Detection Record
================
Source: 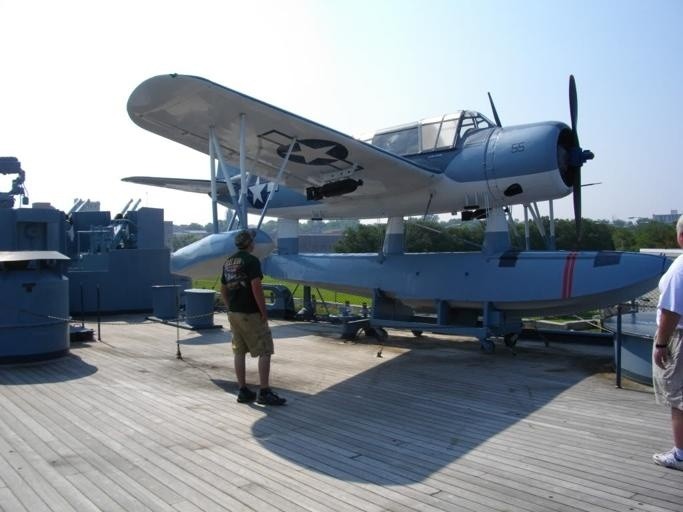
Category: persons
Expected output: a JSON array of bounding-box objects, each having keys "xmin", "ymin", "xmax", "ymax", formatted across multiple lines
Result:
[
  {"xmin": 220, "ymin": 231, "xmax": 287, "ymax": 407},
  {"xmin": 651, "ymin": 214, "xmax": 682, "ymax": 470},
  {"xmin": 344, "ymin": 301, "xmax": 353, "ymax": 315},
  {"xmin": 359, "ymin": 302, "xmax": 371, "ymax": 315}
]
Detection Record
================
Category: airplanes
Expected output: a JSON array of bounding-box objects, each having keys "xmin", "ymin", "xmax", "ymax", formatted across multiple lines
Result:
[{"xmin": 119, "ymin": 70, "xmax": 676, "ymax": 358}]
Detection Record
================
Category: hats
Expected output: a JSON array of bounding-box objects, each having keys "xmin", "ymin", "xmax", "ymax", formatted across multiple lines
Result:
[{"xmin": 234, "ymin": 229, "xmax": 256, "ymax": 249}]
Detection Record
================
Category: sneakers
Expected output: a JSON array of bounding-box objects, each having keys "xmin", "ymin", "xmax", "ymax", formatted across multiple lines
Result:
[
  {"xmin": 652, "ymin": 447, "xmax": 683, "ymax": 471},
  {"xmin": 237, "ymin": 389, "xmax": 257, "ymax": 403},
  {"xmin": 256, "ymin": 391, "xmax": 287, "ymax": 406}
]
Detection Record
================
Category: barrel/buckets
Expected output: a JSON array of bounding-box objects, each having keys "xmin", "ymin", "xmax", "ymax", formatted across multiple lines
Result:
[
  {"xmin": 183, "ymin": 288, "xmax": 216, "ymax": 328},
  {"xmin": 151, "ymin": 285, "xmax": 182, "ymax": 320}
]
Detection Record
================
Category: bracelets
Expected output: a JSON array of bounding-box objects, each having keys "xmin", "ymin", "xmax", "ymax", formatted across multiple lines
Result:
[{"xmin": 655, "ymin": 344, "xmax": 668, "ymax": 348}]
